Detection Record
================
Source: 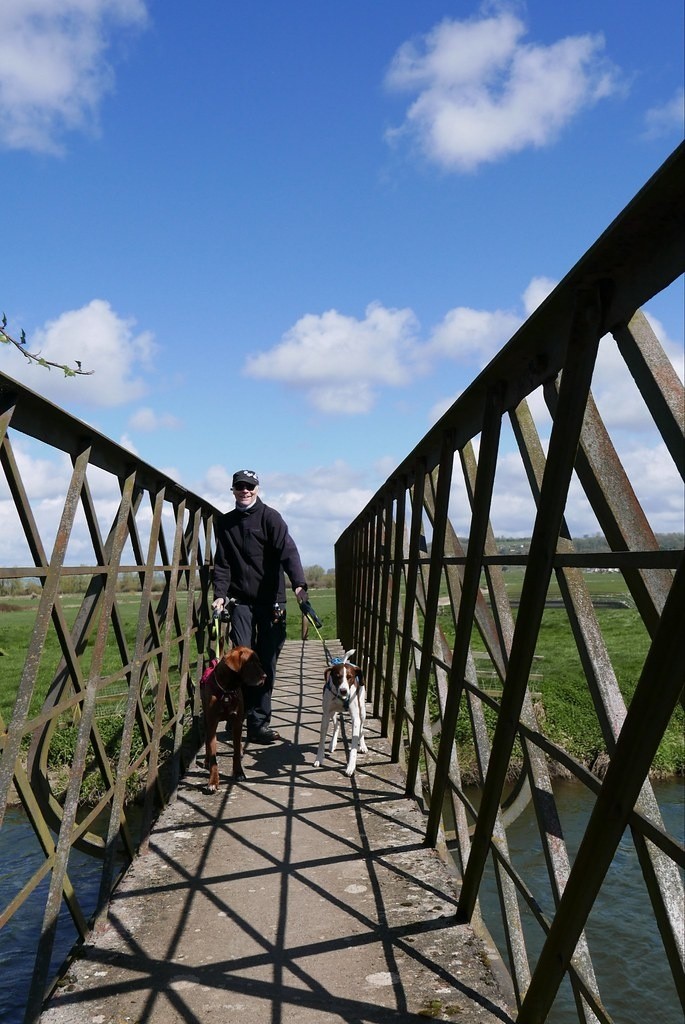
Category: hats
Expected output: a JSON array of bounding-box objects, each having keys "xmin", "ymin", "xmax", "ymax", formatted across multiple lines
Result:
[{"xmin": 232, "ymin": 470, "xmax": 259, "ymax": 485}]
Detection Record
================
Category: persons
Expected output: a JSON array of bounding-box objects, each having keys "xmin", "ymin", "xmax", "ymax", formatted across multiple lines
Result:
[{"xmin": 212, "ymin": 470, "xmax": 309, "ymax": 739}]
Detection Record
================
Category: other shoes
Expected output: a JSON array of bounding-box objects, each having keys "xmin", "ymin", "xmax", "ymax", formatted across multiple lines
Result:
[
  {"xmin": 225, "ymin": 721, "xmax": 235, "ymax": 733},
  {"xmin": 246, "ymin": 723, "xmax": 280, "ymax": 744}
]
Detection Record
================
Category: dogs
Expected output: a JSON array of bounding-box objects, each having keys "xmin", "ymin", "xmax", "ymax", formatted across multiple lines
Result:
[
  {"xmin": 313, "ymin": 649, "xmax": 369, "ymax": 777},
  {"xmin": 200, "ymin": 646, "xmax": 268, "ymax": 793}
]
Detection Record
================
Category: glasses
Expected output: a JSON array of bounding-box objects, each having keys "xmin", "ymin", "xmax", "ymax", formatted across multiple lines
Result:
[{"xmin": 233, "ymin": 483, "xmax": 256, "ymax": 491}]
